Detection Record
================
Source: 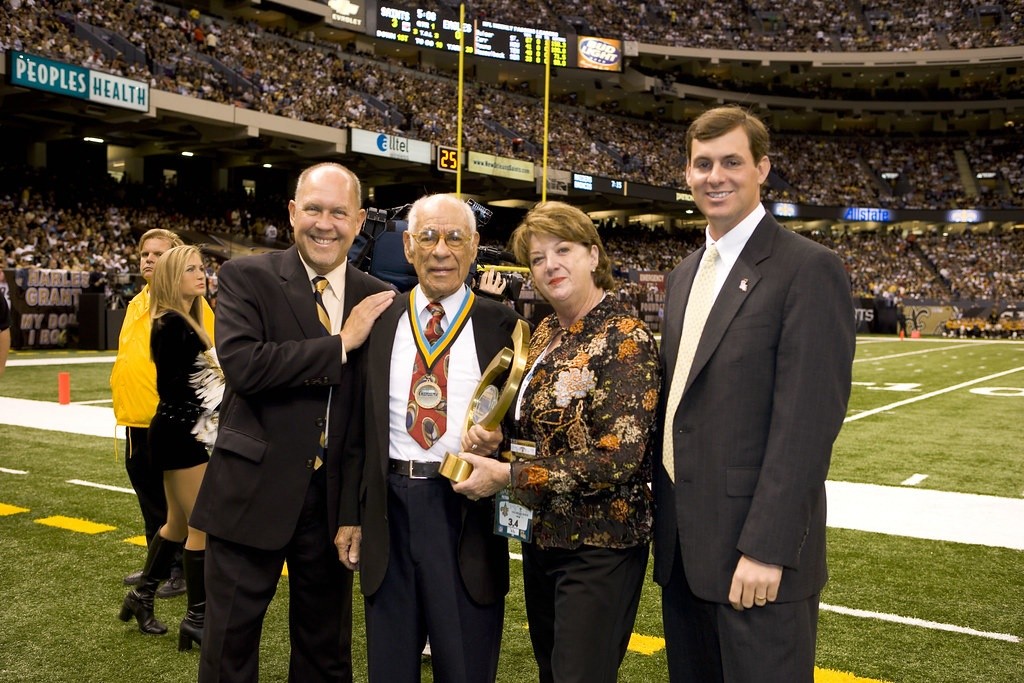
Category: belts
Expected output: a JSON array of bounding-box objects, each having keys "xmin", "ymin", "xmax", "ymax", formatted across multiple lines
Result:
[{"xmin": 388, "ymin": 459, "xmax": 444, "ymax": 480}]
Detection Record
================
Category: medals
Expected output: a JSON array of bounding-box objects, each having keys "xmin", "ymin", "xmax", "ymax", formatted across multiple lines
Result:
[{"xmin": 414, "ymin": 379, "xmax": 442, "ymax": 409}]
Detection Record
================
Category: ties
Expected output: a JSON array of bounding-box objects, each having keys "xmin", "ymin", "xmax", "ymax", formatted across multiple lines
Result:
[
  {"xmin": 662, "ymin": 245, "xmax": 720, "ymax": 483},
  {"xmin": 308, "ymin": 276, "xmax": 331, "ymax": 469},
  {"xmin": 405, "ymin": 301, "xmax": 451, "ymax": 449}
]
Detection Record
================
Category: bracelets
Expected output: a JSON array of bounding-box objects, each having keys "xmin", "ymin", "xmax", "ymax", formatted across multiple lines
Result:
[{"xmin": 508, "ymin": 462, "xmax": 511, "ymax": 488}]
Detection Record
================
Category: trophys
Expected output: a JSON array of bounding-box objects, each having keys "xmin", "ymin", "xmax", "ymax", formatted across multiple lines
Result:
[{"xmin": 437, "ymin": 319, "xmax": 531, "ymax": 482}]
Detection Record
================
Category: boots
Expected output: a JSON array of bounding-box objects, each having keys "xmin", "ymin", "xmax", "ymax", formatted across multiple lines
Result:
[
  {"xmin": 119, "ymin": 524, "xmax": 183, "ymax": 634},
  {"xmin": 178, "ymin": 544, "xmax": 205, "ymax": 651}
]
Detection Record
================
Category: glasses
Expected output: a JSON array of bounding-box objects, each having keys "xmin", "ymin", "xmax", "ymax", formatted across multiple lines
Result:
[{"xmin": 410, "ymin": 229, "xmax": 474, "ymax": 250}]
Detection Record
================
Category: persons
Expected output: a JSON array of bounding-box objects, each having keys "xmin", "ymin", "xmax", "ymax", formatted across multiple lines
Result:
[
  {"xmin": 194, "ymin": 162, "xmax": 400, "ymax": 683},
  {"xmin": 335, "ymin": 189, "xmax": 533, "ymax": 683},
  {"xmin": 110, "ymin": 229, "xmax": 187, "ymax": 599},
  {"xmin": 115, "ymin": 245, "xmax": 226, "ymax": 650},
  {"xmin": 651, "ymin": 108, "xmax": 857, "ymax": 683},
  {"xmin": 448, "ymin": 200, "xmax": 663, "ymax": 683},
  {"xmin": 0, "ymin": 0, "xmax": 1024, "ymax": 377}
]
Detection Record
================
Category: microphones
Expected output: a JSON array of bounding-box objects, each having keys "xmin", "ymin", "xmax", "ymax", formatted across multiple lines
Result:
[{"xmin": 484, "ymin": 247, "xmax": 519, "ymax": 264}]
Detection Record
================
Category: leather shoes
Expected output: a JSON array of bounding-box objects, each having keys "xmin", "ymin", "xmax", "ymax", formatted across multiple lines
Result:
[
  {"xmin": 125, "ymin": 568, "xmax": 145, "ymax": 585},
  {"xmin": 156, "ymin": 577, "xmax": 187, "ymax": 597}
]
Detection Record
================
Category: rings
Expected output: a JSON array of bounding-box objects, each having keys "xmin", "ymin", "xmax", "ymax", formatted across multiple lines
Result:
[
  {"xmin": 754, "ymin": 594, "xmax": 766, "ymax": 601},
  {"xmin": 472, "ymin": 444, "xmax": 477, "ymax": 450}
]
